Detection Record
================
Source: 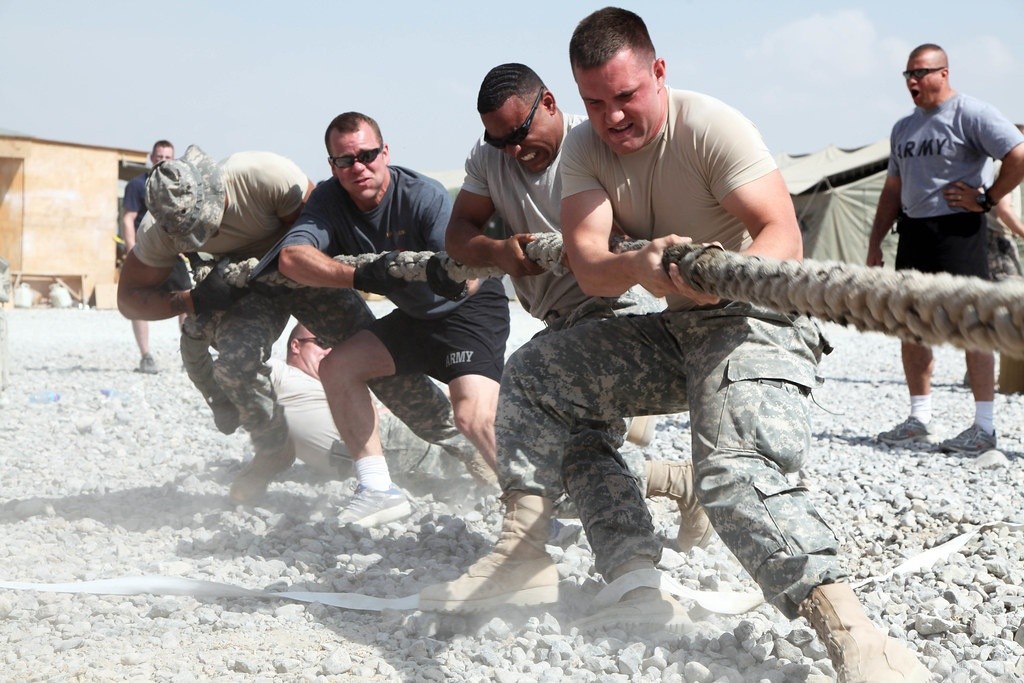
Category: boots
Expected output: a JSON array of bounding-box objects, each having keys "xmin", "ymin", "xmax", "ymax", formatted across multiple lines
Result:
[
  {"xmin": 230, "ymin": 414, "xmax": 298, "ymax": 504},
  {"xmin": 646, "ymin": 461, "xmax": 714, "ymax": 552},
  {"xmin": 188, "ymin": 370, "xmax": 238, "ymax": 436},
  {"xmin": 453, "ymin": 449, "xmax": 502, "ymax": 503},
  {"xmin": 797, "ymin": 583, "xmax": 932, "ymax": 683},
  {"xmin": 419, "ymin": 491, "xmax": 561, "ymax": 616},
  {"xmin": 625, "ymin": 415, "xmax": 659, "ymax": 446},
  {"xmin": 564, "ymin": 555, "xmax": 693, "ymax": 639}
]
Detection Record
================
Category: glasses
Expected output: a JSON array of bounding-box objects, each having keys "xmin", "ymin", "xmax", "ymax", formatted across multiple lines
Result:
[
  {"xmin": 329, "ymin": 145, "xmax": 384, "ymax": 167},
  {"xmin": 902, "ymin": 67, "xmax": 945, "ymax": 80},
  {"xmin": 484, "ymin": 87, "xmax": 544, "ymax": 149},
  {"xmin": 289, "ymin": 336, "xmax": 330, "ymax": 350}
]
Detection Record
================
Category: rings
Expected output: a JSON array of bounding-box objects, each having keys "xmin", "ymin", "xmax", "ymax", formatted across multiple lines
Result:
[{"xmin": 957, "ymin": 194, "xmax": 963, "ymax": 201}]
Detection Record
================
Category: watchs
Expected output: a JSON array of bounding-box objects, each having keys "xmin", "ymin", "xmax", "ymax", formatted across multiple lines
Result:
[{"xmin": 976, "ymin": 185, "xmax": 991, "ymax": 213}]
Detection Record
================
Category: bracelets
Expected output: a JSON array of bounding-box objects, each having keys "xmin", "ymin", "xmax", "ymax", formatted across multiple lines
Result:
[{"xmin": 985, "ymin": 188, "xmax": 1000, "ymax": 207}]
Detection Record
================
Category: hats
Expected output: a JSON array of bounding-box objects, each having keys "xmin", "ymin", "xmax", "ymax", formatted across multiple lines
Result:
[{"xmin": 145, "ymin": 143, "xmax": 225, "ymax": 253}]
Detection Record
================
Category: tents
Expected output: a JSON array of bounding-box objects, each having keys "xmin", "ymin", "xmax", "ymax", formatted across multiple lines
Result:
[{"xmin": 741, "ymin": 140, "xmax": 900, "ymax": 271}]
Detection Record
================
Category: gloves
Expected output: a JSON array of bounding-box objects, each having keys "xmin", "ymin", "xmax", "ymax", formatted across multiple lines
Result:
[
  {"xmin": 354, "ymin": 250, "xmax": 408, "ymax": 296},
  {"xmin": 189, "ymin": 257, "xmax": 251, "ymax": 315},
  {"xmin": 426, "ymin": 251, "xmax": 466, "ymax": 299}
]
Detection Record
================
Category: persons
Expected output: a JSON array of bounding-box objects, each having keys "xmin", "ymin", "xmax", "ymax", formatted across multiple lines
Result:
[
  {"xmin": 866, "ymin": 43, "xmax": 1024, "ymax": 457},
  {"xmin": 419, "ymin": 6, "xmax": 937, "ymax": 682},
  {"xmin": 245, "ymin": 112, "xmax": 510, "ymax": 531},
  {"xmin": 261, "ymin": 319, "xmax": 718, "ymax": 553},
  {"xmin": 444, "ymin": 63, "xmax": 703, "ymax": 638},
  {"xmin": 118, "ymin": 140, "xmax": 316, "ymax": 499}
]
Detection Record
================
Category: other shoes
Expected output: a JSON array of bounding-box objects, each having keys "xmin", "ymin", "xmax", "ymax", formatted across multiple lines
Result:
[{"xmin": 139, "ymin": 352, "xmax": 159, "ymax": 374}]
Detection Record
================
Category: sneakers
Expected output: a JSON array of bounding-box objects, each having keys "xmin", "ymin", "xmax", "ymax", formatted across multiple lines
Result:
[
  {"xmin": 544, "ymin": 517, "xmax": 582, "ymax": 551},
  {"xmin": 337, "ymin": 480, "xmax": 410, "ymax": 528},
  {"xmin": 878, "ymin": 416, "xmax": 938, "ymax": 445},
  {"xmin": 939, "ymin": 425, "xmax": 997, "ymax": 454}
]
